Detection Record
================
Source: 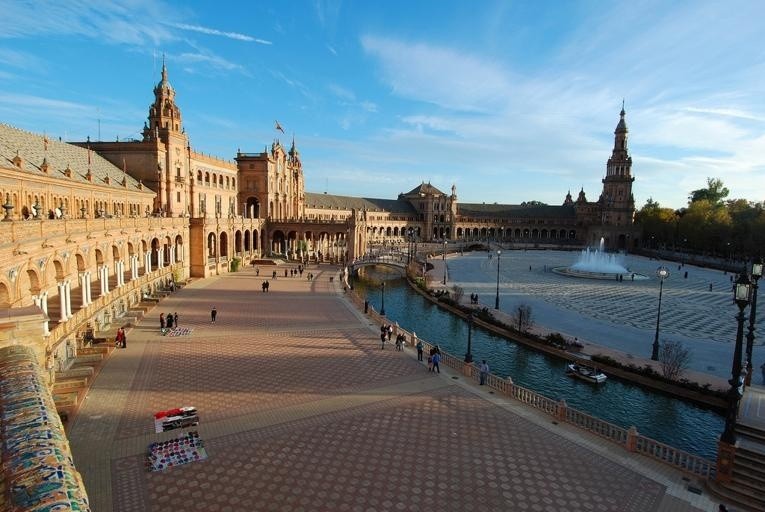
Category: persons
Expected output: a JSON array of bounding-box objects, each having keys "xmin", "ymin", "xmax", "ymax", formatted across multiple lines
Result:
[
  {"xmin": 473, "ymin": 295, "xmax": 479, "ymax": 304},
  {"xmin": 169, "ymin": 281, "xmax": 174, "ymax": 291},
  {"xmin": 574, "ymin": 337, "xmax": 580, "ymax": 346},
  {"xmin": 211, "ymin": 307, "xmax": 217, "ymax": 324},
  {"xmin": 160, "ymin": 312, "xmax": 179, "ymax": 328},
  {"xmin": 256, "ymin": 260, "xmax": 313, "ymax": 293},
  {"xmin": 115, "ymin": 328, "xmax": 122, "ymax": 346},
  {"xmin": 479, "ymin": 360, "xmax": 489, "ymax": 385},
  {"xmin": 529, "ymin": 266, "xmax": 531, "ymax": 272},
  {"xmin": 615, "ymin": 257, "xmax": 739, "ymax": 292},
  {"xmin": 471, "ymin": 293, "xmax": 474, "ymax": 304},
  {"xmin": 573, "ymin": 359, "xmax": 601, "ymax": 376},
  {"xmin": 381, "ymin": 323, "xmax": 441, "ymax": 373},
  {"xmin": 121, "ymin": 327, "xmax": 126, "ymax": 348}
]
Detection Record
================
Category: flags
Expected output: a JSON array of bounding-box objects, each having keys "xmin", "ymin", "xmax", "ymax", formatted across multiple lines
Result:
[{"xmin": 275, "ymin": 121, "xmax": 285, "ymax": 134}]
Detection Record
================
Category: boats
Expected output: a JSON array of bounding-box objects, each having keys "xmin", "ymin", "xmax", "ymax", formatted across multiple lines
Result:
[{"xmin": 566, "ymin": 360, "xmax": 609, "ymax": 384}]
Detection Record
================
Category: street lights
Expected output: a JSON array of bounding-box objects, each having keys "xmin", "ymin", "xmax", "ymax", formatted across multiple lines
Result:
[
  {"xmin": 444, "ymin": 241, "xmax": 447, "ymax": 285},
  {"xmin": 501, "ymin": 226, "xmax": 504, "ymax": 247},
  {"xmin": 570, "ymin": 231, "xmax": 574, "ymax": 251},
  {"xmin": 494, "ymin": 249, "xmax": 504, "ymax": 309},
  {"xmin": 465, "ymin": 304, "xmax": 475, "ymax": 365},
  {"xmin": 680, "ymin": 233, "xmax": 688, "ymax": 266},
  {"xmin": 460, "ymin": 231, "xmax": 464, "ymax": 257},
  {"xmin": 651, "ymin": 266, "xmax": 669, "ymax": 362},
  {"xmin": 747, "ymin": 248, "xmax": 763, "ymax": 372},
  {"xmin": 624, "ymin": 234, "xmax": 630, "ymax": 256},
  {"xmin": 406, "ymin": 227, "xmax": 419, "ymax": 262},
  {"xmin": 649, "ymin": 234, "xmax": 656, "ymax": 260},
  {"xmin": 525, "ymin": 231, "xmax": 528, "ymax": 251},
  {"xmin": 442, "ymin": 232, "xmax": 446, "ymax": 260},
  {"xmin": 725, "ymin": 237, "xmax": 732, "ymax": 274},
  {"xmin": 379, "ymin": 282, "xmax": 388, "ymax": 315},
  {"xmin": 720, "ymin": 261, "xmax": 755, "ymax": 444},
  {"xmin": 486, "ymin": 227, "xmax": 490, "ymax": 252}
]
